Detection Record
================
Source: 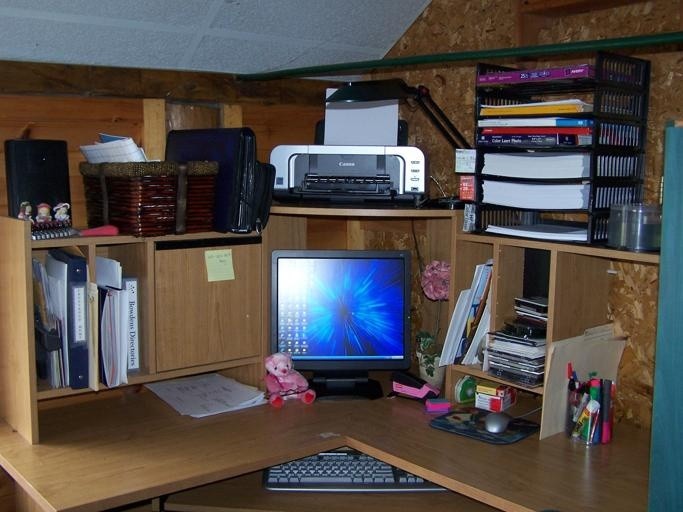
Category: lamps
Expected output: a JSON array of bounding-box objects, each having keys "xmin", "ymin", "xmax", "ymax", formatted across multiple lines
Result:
[{"xmin": 325, "ymin": 77, "xmax": 472, "ymax": 149}]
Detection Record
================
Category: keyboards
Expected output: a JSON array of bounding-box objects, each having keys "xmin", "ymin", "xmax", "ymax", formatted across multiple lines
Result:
[{"xmin": 261, "ymin": 452, "xmax": 447, "ymax": 491}]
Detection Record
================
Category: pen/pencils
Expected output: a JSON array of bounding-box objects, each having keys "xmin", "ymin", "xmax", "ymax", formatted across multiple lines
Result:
[{"xmin": 567, "ymin": 363, "xmax": 615, "ymax": 444}]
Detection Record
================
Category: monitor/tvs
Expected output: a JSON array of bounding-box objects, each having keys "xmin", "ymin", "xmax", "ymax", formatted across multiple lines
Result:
[{"xmin": 272, "ymin": 249, "xmax": 411, "ymax": 399}]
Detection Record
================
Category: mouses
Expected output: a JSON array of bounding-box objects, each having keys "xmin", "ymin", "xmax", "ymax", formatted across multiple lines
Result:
[{"xmin": 485, "ymin": 412, "xmax": 512, "ymax": 434}]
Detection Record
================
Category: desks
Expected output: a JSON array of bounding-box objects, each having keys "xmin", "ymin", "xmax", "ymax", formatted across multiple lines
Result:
[{"xmin": 0, "ymin": 373, "xmax": 651, "ymax": 512}]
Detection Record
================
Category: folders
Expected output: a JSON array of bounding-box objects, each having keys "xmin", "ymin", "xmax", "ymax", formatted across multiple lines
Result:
[{"xmin": 49, "ymin": 248, "xmax": 86, "ymax": 388}]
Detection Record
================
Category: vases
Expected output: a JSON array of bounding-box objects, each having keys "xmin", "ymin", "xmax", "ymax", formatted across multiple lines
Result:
[{"xmin": 416, "ymin": 351, "xmax": 443, "ymax": 390}]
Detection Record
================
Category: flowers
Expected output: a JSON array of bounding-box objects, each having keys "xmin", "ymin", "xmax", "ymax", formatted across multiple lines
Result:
[{"xmin": 415, "ymin": 258, "xmax": 451, "ymax": 378}]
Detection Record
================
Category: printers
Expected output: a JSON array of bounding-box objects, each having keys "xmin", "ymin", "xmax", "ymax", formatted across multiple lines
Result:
[{"xmin": 269, "ymin": 118, "xmax": 430, "ymax": 209}]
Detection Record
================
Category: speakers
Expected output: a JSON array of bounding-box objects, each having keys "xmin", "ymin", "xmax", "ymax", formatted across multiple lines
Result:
[{"xmin": 3, "ymin": 138, "xmax": 73, "ymax": 228}]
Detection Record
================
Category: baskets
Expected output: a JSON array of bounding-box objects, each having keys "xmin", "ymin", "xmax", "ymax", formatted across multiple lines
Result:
[{"xmin": 80, "ymin": 160, "xmax": 218, "ymax": 238}]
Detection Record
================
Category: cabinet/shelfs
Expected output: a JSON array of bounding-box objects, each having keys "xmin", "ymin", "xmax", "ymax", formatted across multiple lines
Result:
[
  {"xmin": 444, "ymin": 231, "xmax": 610, "ymax": 430},
  {"xmin": 2, "ymin": 215, "xmax": 152, "ymax": 400},
  {"xmin": 152, "ymin": 241, "xmax": 262, "ymax": 383}
]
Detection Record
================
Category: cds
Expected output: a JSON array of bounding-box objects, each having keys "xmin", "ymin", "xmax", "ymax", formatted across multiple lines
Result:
[{"xmin": 607, "ymin": 219, "xmax": 661, "ymax": 250}]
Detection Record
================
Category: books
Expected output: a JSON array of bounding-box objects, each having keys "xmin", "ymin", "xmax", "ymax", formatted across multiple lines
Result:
[
  {"xmin": 30, "ymin": 227, "xmax": 73, "ymax": 240},
  {"xmin": 438, "ymin": 258, "xmax": 547, "ymax": 389},
  {"xmin": 144, "ymin": 371, "xmax": 268, "ymax": 419},
  {"xmin": 78, "ymin": 131, "xmax": 161, "ymax": 163},
  {"xmin": 32, "ymin": 245, "xmax": 141, "ymax": 392},
  {"xmin": 477, "ymin": 97, "xmax": 639, "ymax": 242}
]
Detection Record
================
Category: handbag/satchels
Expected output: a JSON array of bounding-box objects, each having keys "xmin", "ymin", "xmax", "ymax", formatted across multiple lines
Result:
[
  {"xmin": 254, "ymin": 159, "xmax": 276, "ymax": 230},
  {"xmin": 164, "ymin": 127, "xmax": 255, "ymax": 234}
]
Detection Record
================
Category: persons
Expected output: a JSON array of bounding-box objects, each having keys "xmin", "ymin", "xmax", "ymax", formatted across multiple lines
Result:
[
  {"xmin": 17, "ymin": 198, "xmax": 35, "ymax": 224},
  {"xmin": 35, "ymin": 201, "xmax": 52, "ymax": 228},
  {"xmin": 53, "ymin": 201, "xmax": 72, "ymax": 227}
]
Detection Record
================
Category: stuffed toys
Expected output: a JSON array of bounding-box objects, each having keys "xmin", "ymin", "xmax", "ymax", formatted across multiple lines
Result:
[{"xmin": 263, "ymin": 351, "xmax": 316, "ymax": 407}]
